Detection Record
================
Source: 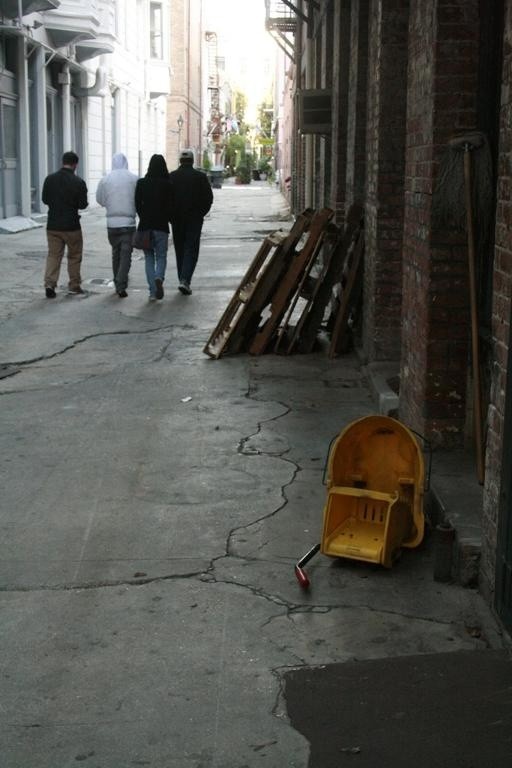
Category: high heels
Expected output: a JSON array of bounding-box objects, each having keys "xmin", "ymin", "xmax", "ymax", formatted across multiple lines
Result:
[{"xmin": 116, "ymin": 289, "xmax": 127, "ymax": 297}]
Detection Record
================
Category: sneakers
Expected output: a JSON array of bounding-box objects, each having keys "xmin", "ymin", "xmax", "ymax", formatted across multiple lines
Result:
[
  {"xmin": 68, "ymin": 286, "xmax": 83, "ymax": 294},
  {"xmin": 177, "ymin": 285, "xmax": 192, "ymax": 295},
  {"xmin": 147, "ymin": 277, "xmax": 164, "ymax": 302},
  {"xmin": 45, "ymin": 288, "xmax": 56, "ymax": 298}
]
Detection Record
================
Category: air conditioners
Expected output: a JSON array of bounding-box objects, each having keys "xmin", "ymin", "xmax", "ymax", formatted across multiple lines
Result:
[{"xmin": 295, "ymin": 88, "xmax": 331, "ymax": 135}]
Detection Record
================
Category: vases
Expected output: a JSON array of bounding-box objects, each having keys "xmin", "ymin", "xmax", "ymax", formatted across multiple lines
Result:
[{"xmin": 253, "ymin": 170, "xmax": 261, "ymax": 180}]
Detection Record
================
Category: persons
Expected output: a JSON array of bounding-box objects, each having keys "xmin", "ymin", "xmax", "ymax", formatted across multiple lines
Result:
[
  {"xmin": 40, "ymin": 150, "xmax": 88, "ymax": 298},
  {"xmin": 94, "ymin": 153, "xmax": 139, "ymax": 298},
  {"xmin": 134, "ymin": 153, "xmax": 171, "ymax": 301},
  {"xmin": 162, "ymin": 149, "xmax": 215, "ymax": 294}
]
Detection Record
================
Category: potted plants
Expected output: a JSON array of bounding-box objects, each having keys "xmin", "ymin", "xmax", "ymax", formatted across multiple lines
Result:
[
  {"xmin": 235, "ymin": 155, "xmax": 251, "ymax": 184},
  {"xmin": 259, "ymin": 154, "xmax": 271, "ymax": 181}
]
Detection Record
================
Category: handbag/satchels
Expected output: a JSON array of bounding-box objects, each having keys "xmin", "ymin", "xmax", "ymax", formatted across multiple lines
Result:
[{"xmin": 131, "ymin": 231, "xmax": 150, "ymax": 249}]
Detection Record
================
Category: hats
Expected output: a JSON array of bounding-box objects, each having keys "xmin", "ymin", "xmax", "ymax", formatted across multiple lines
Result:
[{"xmin": 179, "ymin": 150, "xmax": 193, "ymax": 160}]
